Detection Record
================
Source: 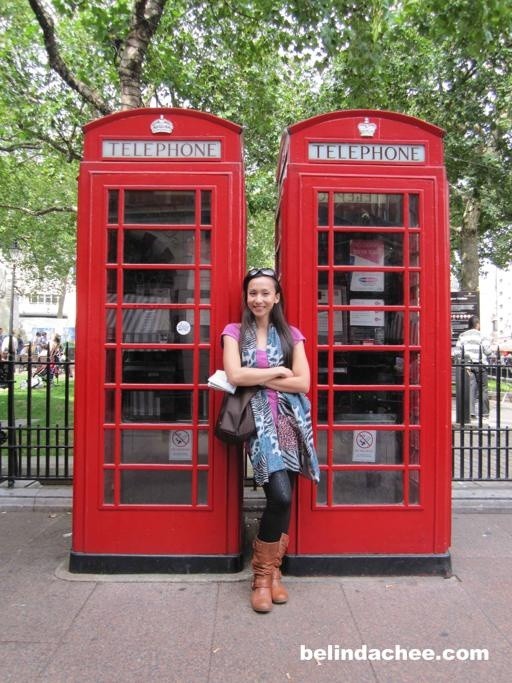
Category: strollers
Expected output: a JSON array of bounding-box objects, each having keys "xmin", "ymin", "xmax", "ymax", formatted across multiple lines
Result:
[{"xmin": 31, "ymin": 351, "xmax": 60, "ymax": 386}]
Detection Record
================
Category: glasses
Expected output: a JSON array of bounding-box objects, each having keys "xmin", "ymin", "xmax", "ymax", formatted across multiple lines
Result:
[{"xmin": 248, "ymin": 268, "xmax": 275, "ymax": 278}]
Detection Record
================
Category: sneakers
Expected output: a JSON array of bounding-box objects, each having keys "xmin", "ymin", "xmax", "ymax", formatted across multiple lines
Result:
[{"xmin": 470, "ymin": 415, "xmax": 490, "ymax": 429}]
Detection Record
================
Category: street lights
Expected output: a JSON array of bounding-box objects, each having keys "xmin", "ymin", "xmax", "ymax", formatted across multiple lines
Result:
[{"xmin": 9, "ymin": 237, "xmax": 26, "ymax": 357}]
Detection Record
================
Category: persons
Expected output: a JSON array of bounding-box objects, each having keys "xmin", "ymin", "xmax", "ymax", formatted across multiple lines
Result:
[
  {"xmin": 0, "ymin": 327, "xmax": 71, "ymax": 387},
  {"xmin": 456, "ymin": 316, "xmax": 492, "ymax": 420},
  {"xmin": 220, "ymin": 268, "xmax": 312, "ymax": 613}
]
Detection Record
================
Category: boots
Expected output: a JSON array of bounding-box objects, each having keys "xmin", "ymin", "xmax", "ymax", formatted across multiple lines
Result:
[
  {"xmin": 250, "ymin": 535, "xmax": 280, "ymax": 612},
  {"xmin": 270, "ymin": 533, "xmax": 290, "ymax": 604}
]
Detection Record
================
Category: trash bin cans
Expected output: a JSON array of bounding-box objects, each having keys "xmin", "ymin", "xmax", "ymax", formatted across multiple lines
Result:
[
  {"xmin": 167, "ymin": 420, "xmax": 208, "ymax": 504},
  {"xmin": 336, "ymin": 413, "xmax": 397, "ymax": 505}
]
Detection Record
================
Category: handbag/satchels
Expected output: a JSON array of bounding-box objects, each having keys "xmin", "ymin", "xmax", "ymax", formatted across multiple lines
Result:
[{"xmin": 213, "ymin": 385, "xmax": 261, "ymax": 444}]
